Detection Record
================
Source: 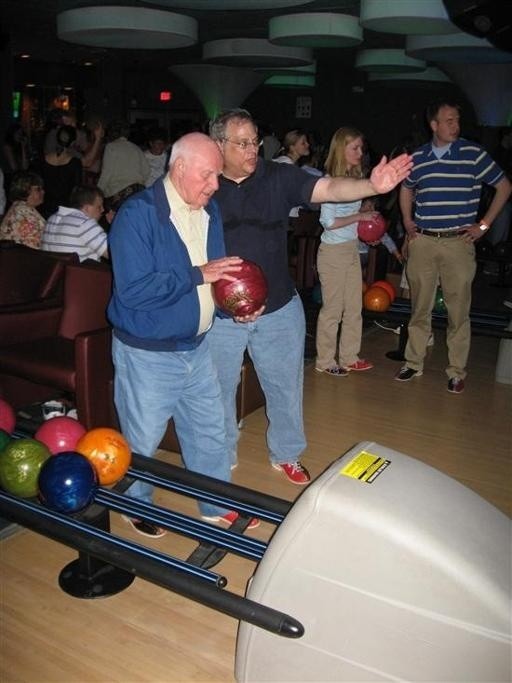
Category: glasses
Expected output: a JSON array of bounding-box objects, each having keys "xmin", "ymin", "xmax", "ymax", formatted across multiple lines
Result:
[{"xmin": 221, "ymin": 135, "xmax": 261, "ymax": 148}]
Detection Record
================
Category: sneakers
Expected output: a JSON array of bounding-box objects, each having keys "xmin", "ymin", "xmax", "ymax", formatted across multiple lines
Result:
[
  {"xmin": 120, "ymin": 512, "xmax": 169, "ymax": 539},
  {"xmin": 314, "ymin": 362, "xmax": 350, "ymax": 377},
  {"xmin": 372, "ymin": 317, "xmax": 404, "ymax": 335},
  {"xmin": 340, "ymin": 357, "xmax": 376, "ymax": 371},
  {"xmin": 425, "ymin": 331, "xmax": 435, "ymax": 347},
  {"xmin": 201, "ymin": 507, "xmax": 262, "ymax": 530},
  {"xmin": 392, "ymin": 365, "xmax": 424, "ymax": 382},
  {"xmin": 446, "ymin": 374, "xmax": 465, "ymax": 394},
  {"xmin": 270, "ymin": 458, "xmax": 312, "ymax": 484}
]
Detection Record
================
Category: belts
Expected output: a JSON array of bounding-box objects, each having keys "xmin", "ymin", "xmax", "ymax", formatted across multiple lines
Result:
[{"xmin": 413, "ymin": 224, "xmax": 469, "ymax": 239}]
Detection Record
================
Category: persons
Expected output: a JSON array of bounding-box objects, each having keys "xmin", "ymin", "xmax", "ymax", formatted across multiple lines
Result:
[{"xmin": 1, "ymin": 99, "xmax": 511, "ymax": 540}]
